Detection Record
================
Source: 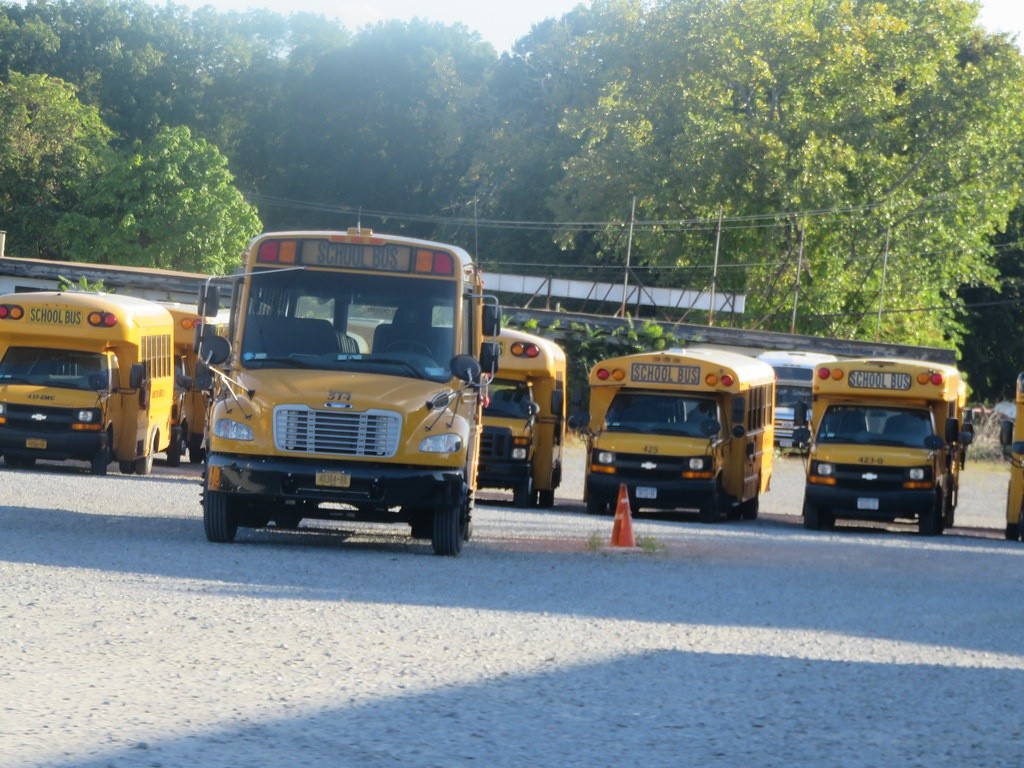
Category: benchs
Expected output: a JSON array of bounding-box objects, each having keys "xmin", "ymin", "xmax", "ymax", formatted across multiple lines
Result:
[
  {"xmin": 17, "ymin": 355, "xmax": 77, "ymax": 376},
  {"xmin": 243, "ymin": 315, "xmax": 360, "ymax": 355}
]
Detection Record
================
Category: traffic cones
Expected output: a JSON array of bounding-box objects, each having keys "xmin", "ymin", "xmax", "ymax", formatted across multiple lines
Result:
[{"xmin": 611, "ymin": 482, "xmax": 635, "ymax": 548}]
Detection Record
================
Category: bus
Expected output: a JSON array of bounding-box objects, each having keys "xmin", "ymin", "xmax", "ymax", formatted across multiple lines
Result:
[
  {"xmin": 755, "ymin": 350, "xmax": 838, "ymax": 450},
  {"xmin": 473, "ymin": 326, "xmax": 566, "ymax": 508},
  {"xmin": 582, "ymin": 347, "xmax": 778, "ymax": 523},
  {"xmin": 193, "ymin": 228, "xmax": 502, "ymax": 557},
  {"xmin": 998, "ymin": 374, "xmax": 1024, "ymax": 542},
  {"xmin": 0, "ymin": 291, "xmax": 174, "ymax": 476},
  {"xmin": 801, "ymin": 359, "xmax": 967, "ymax": 536},
  {"xmin": 150, "ymin": 302, "xmax": 228, "ymax": 463}
]
